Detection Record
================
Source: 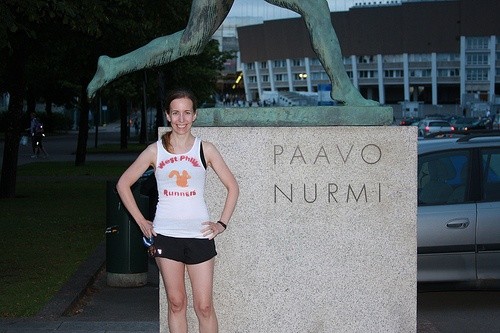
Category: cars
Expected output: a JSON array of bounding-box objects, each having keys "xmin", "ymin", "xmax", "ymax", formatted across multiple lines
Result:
[
  {"xmin": 417, "ymin": 130, "xmax": 500, "ymax": 293},
  {"xmin": 410, "ymin": 118, "xmax": 454, "ymax": 139}
]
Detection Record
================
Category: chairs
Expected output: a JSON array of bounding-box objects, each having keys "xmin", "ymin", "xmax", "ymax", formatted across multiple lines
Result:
[{"xmin": 418, "ymin": 156, "xmax": 470, "ymax": 205}]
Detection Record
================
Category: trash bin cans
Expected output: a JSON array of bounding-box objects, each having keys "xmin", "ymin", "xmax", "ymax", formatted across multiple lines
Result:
[{"xmin": 103, "ymin": 166, "xmax": 158, "ymax": 288}]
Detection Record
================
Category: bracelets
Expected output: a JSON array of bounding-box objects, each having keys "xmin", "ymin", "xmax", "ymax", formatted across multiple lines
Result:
[{"xmin": 217, "ymin": 221, "xmax": 227, "ymax": 229}]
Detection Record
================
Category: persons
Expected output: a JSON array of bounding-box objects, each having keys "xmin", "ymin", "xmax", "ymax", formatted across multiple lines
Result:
[
  {"xmin": 213, "ymin": 91, "xmax": 278, "ymax": 109},
  {"xmin": 26, "ymin": 117, "xmax": 50, "ymax": 159},
  {"xmin": 115, "ymin": 90, "xmax": 239, "ymax": 333},
  {"xmin": 133, "ymin": 117, "xmax": 141, "ymax": 136},
  {"xmin": 88, "ymin": 1, "xmax": 379, "ymax": 106}
]
